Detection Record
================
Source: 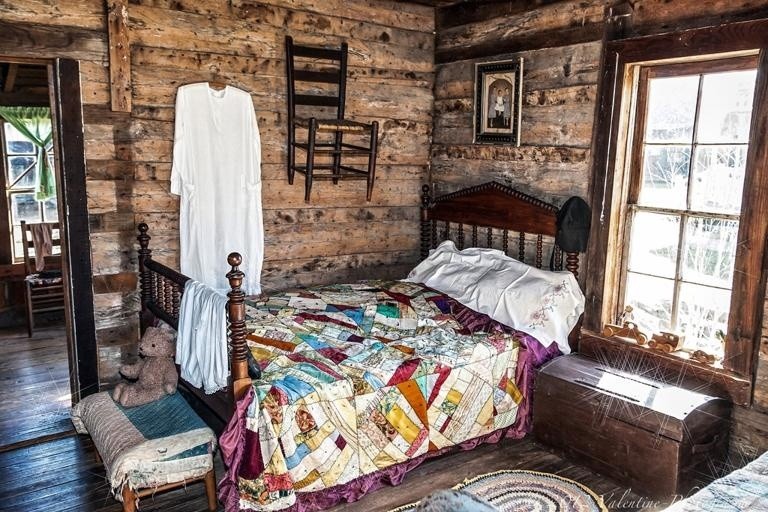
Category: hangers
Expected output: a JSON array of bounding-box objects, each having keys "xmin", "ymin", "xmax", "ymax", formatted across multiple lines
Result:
[{"xmin": 173, "ymin": 63, "xmax": 251, "ymax": 100}]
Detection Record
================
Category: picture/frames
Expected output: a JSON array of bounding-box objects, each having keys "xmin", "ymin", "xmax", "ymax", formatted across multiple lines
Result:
[{"xmin": 472, "ymin": 58, "xmax": 525, "ymax": 146}]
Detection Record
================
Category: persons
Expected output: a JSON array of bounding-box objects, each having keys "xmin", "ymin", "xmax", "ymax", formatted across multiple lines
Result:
[{"xmin": 494, "ymin": 88, "xmax": 511, "ymax": 129}]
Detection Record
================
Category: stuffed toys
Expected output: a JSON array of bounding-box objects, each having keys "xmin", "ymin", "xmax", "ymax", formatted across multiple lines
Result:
[{"xmin": 113, "ymin": 325, "xmax": 178, "ymax": 408}]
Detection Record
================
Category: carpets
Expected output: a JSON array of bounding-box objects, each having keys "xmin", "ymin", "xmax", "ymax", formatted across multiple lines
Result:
[{"xmin": 392, "ymin": 470, "xmax": 609, "ymax": 512}]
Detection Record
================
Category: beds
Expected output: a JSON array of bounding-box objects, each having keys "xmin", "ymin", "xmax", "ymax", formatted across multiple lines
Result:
[{"xmin": 119, "ymin": 181, "xmax": 593, "ymax": 512}]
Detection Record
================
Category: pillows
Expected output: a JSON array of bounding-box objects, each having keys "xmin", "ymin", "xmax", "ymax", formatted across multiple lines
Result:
[{"xmin": 398, "ymin": 239, "xmax": 586, "ymax": 354}]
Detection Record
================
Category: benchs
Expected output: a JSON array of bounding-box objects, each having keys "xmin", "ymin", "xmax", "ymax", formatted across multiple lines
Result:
[{"xmin": 73, "ymin": 383, "xmax": 217, "ymax": 511}]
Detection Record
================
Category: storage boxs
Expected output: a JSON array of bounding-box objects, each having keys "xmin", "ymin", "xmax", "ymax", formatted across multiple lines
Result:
[{"xmin": 533, "ymin": 353, "xmax": 733, "ymax": 502}]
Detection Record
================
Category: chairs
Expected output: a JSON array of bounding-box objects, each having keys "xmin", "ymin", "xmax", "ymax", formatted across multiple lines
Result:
[
  {"xmin": 20, "ymin": 220, "xmax": 66, "ymax": 337},
  {"xmin": 284, "ymin": 36, "xmax": 380, "ymax": 202}
]
